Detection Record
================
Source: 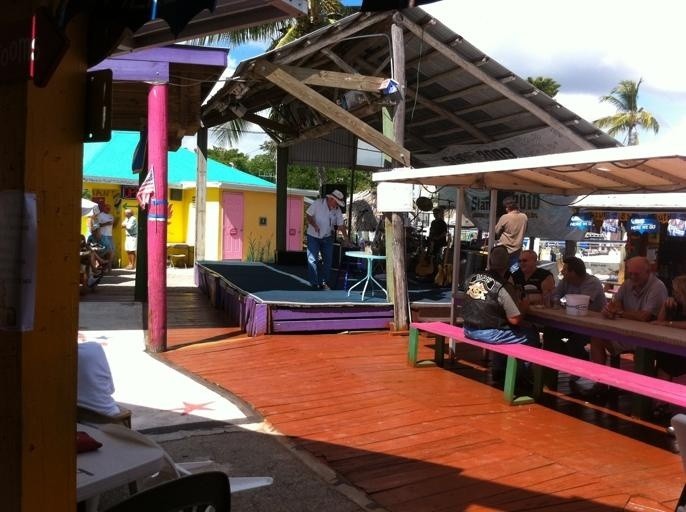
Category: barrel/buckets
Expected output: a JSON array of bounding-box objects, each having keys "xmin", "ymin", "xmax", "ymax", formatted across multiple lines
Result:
[{"xmin": 562, "ymin": 294, "xmax": 591, "ymax": 316}]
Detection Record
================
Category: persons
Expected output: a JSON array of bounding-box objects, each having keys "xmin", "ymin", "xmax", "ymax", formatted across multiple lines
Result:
[
  {"xmin": 427, "ymin": 208, "xmax": 448, "ymax": 265},
  {"xmin": 121, "ymin": 208, "xmax": 138, "ymax": 270},
  {"xmin": 80, "ymin": 205, "xmax": 115, "ymax": 296},
  {"xmin": 305, "ymin": 189, "xmax": 353, "ymax": 291},
  {"xmin": 494, "ymin": 195, "xmax": 529, "ymax": 268}
]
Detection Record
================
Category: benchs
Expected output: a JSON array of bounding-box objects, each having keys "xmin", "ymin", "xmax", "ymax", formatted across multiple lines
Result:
[{"xmin": 405, "ymin": 319, "xmax": 686, "ymax": 437}]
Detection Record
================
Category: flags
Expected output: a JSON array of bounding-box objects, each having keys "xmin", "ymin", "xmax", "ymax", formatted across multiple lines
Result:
[{"xmin": 136, "ymin": 165, "xmax": 156, "ymax": 210}]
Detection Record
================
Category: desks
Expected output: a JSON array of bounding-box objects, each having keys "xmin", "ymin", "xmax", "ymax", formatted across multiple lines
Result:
[
  {"xmin": 342, "ymin": 249, "xmax": 390, "ymax": 302},
  {"xmin": 451, "ymin": 289, "xmax": 686, "ymax": 410},
  {"xmin": 72, "ymin": 419, "xmax": 166, "ymax": 511}
]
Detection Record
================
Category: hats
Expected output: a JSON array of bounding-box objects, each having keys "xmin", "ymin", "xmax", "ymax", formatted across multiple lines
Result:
[{"xmin": 326, "ymin": 190, "xmax": 346, "ymax": 208}]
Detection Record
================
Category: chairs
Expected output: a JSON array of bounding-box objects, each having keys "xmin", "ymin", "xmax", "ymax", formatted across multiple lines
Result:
[
  {"xmin": 101, "ymin": 466, "xmax": 232, "ymax": 512},
  {"xmin": 166, "ymin": 243, "xmax": 190, "ymax": 272},
  {"xmin": 75, "ymin": 339, "xmax": 141, "ymax": 497}
]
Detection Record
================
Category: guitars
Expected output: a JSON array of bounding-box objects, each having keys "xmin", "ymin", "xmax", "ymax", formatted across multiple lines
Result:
[
  {"xmin": 434, "ymin": 233, "xmax": 453, "ymax": 286},
  {"xmin": 415, "ymin": 237, "xmax": 435, "ymax": 276}
]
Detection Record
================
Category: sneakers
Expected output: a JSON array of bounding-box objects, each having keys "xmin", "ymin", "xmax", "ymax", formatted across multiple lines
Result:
[{"xmin": 320, "ymin": 284, "xmax": 333, "ymax": 290}]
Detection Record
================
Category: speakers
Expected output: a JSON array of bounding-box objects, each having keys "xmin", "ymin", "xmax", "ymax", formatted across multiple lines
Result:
[
  {"xmin": 82, "ymin": 69, "xmax": 113, "ymax": 142},
  {"xmin": 319, "ymin": 184, "xmax": 347, "ymax": 214}
]
